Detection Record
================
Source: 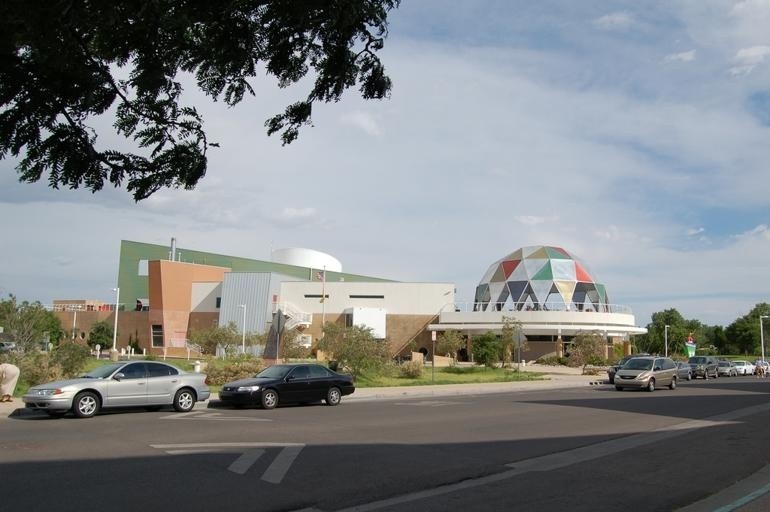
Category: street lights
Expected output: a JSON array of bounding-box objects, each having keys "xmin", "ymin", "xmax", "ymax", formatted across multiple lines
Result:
[
  {"xmin": 759, "ymin": 314, "xmax": 769, "ymax": 361},
  {"xmin": 112, "ymin": 288, "xmax": 120, "ymax": 350},
  {"xmin": 70, "ymin": 305, "xmax": 82, "ymax": 342},
  {"xmin": 237, "ymin": 304, "xmax": 247, "ymax": 354},
  {"xmin": 664, "ymin": 324, "xmax": 671, "ymax": 357}
]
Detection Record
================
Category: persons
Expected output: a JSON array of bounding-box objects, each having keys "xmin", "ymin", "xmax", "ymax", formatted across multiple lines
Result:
[
  {"xmin": 1, "ymin": 362, "xmax": 22, "ymax": 402},
  {"xmin": 754, "ymin": 356, "xmax": 766, "ymax": 379}
]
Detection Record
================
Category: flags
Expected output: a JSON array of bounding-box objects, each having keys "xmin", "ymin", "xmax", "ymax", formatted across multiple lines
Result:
[{"xmin": 314, "ymin": 270, "xmax": 325, "ymax": 282}]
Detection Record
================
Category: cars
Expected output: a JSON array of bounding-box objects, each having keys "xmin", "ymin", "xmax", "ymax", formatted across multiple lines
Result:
[
  {"xmin": 606, "ymin": 352, "xmax": 770, "ymax": 391},
  {"xmin": 0, "ymin": 341, "xmax": 24, "ymax": 353},
  {"xmin": 22, "ymin": 359, "xmax": 212, "ymax": 420},
  {"xmin": 219, "ymin": 361, "xmax": 355, "ymax": 409}
]
